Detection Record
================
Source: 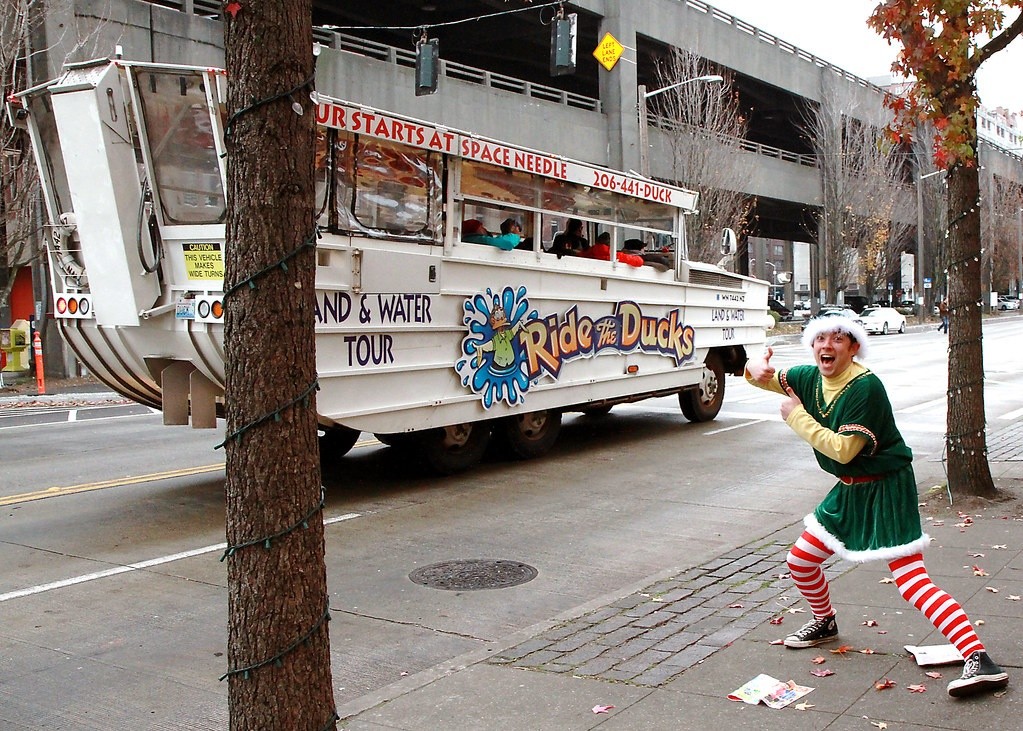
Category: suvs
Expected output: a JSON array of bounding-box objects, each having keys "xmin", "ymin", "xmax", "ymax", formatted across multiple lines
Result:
[{"xmin": 998, "ymin": 295, "xmax": 1020, "ymax": 307}]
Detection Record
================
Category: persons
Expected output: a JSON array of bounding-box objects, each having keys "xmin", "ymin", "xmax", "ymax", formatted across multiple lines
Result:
[
  {"xmin": 936, "ymin": 297, "xmax": 950, "ymax": 335},
  {"xmin": 499, "ymin": 218, "xmax": 534, "ymax": 252},
  {"xmin": 742, "ymin": 315, "xmax": 1009, "ymax": 699},
  {"xmin": 623, "ymin": 239, "xmax": 647, "ymax": 253},
  {"xmin": 580, "ymin": 231, "xmax": 643, "ymax": 269},
  {"xmin": 552, "ymin": 218, "xmax": 591, "ymax": 258},
  {"xmin": 462, "ymin": 218, "xmax": 524, "ymax": 251}
]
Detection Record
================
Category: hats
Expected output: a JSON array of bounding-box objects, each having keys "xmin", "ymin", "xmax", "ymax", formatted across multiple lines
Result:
[
  {"xmin": 501, "ymin": 218, "xmax": 515, "ymax": 231},
  {"xmin": 803, "ymin": 310, "xmax": 870, "ymax": 360},
  {"xmin": 624, "ymin": 239, "xmax": 647, "ymax": 250},
  {"xmin": 462, "ymin": 219, "xmax": 483, "ymax": 234}
]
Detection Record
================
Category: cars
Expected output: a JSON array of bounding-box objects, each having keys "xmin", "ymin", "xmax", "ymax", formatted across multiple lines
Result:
[
  {"xmin": 801, "ymin": 304, "xmax": 864, "ymax": 331},
  {"xmin": 997, "ymin": 299, "xmax": 1018, "ymax": 311},
  {"xmin": 770, "ymin": 298, "xmax": 941, "ymax": 319},
  {"xmin": 859, "ymin": 307, "xmax": 906, "ymax": 335}
]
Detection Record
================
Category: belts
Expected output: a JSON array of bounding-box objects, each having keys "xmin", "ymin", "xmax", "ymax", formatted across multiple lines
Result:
[{"xmin": 837, "ymin": 474, "xmax": 886, "ymax": 485}]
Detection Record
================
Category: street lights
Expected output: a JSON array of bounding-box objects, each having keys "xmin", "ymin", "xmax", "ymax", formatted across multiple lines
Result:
[{"xmin": 637, "ymin": 75, "xmax": 723, "ymax": 178}]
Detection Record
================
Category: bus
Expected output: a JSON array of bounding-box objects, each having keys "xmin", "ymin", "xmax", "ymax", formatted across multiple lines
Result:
[{"xmin": 5, "ymin": 56, "xmax": 794, "ymax": 473}]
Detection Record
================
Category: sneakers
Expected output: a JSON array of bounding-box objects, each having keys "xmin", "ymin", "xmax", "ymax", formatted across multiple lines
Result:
[
  {"xmin": 783, "ymin": 608, "xmax": 838, "ymax": 647},
  {"xmin": 947, "ymin": 652, "xmax": 1010, "ymax": 695}
]
